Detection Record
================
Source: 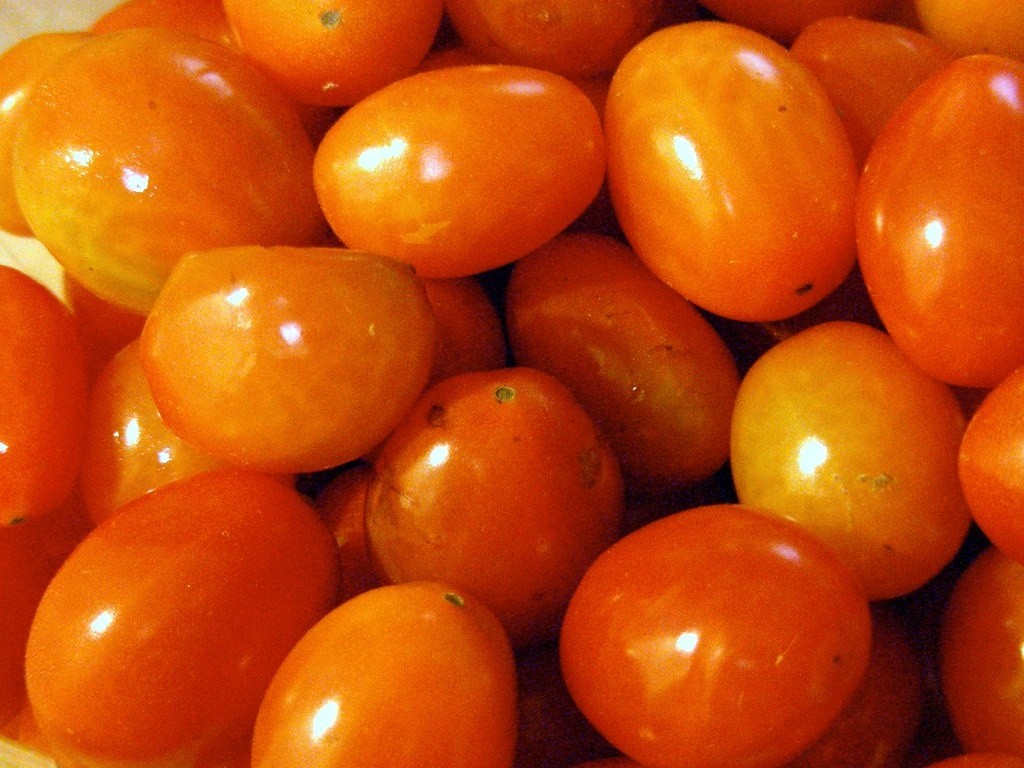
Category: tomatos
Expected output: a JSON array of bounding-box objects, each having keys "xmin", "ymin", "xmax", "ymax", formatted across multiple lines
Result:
[{"xmin": 0, "ymin": 0, "xmax": 1024, "ymax": 768}]
